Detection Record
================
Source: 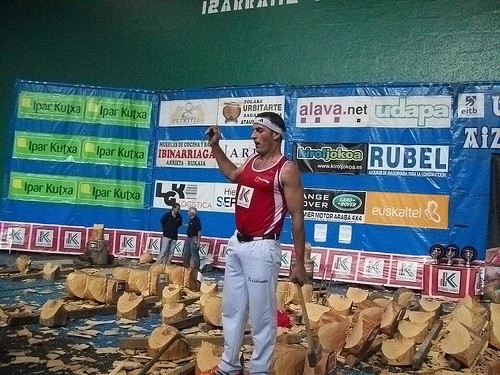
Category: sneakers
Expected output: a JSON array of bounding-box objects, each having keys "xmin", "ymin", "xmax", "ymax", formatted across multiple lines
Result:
[{"xmin": 197, "ymin": 365, "xmax": 240, "ymax": 375}]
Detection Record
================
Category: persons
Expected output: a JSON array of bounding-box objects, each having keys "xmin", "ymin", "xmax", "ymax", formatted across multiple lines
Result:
[
  {"xmin": 204, "ymin": 112, "xmax": 306, "ymax": 375},
  {"xmin": 156, "ymin": 203, "xmax": 183, "ymax": 263},
  {"xmin": 182, "ymin": 207, "xmax": 201, "ymax": 268}
]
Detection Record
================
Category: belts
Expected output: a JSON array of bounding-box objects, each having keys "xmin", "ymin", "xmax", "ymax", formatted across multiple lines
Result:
[{"xmin": 237, "ymin": 233, "xmax": 279, "ymax": 242}]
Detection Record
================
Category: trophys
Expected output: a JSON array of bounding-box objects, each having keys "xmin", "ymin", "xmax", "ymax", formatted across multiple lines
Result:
[
  {"xmin": 446, "ymin": 247, "xmax": 456, "ymax": 266},
  {"xmin": 431, "ymin": 251, "xmax": 438, "ymax": 265},
  {"xmin": 464, "ymin": 249, "xmax": 473, "ymax": 268}
]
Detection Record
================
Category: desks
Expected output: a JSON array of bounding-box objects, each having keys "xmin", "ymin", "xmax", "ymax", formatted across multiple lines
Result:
[{"xmin": 422, "ymin": 261, "xmax": 481, "ymax": 302}]
